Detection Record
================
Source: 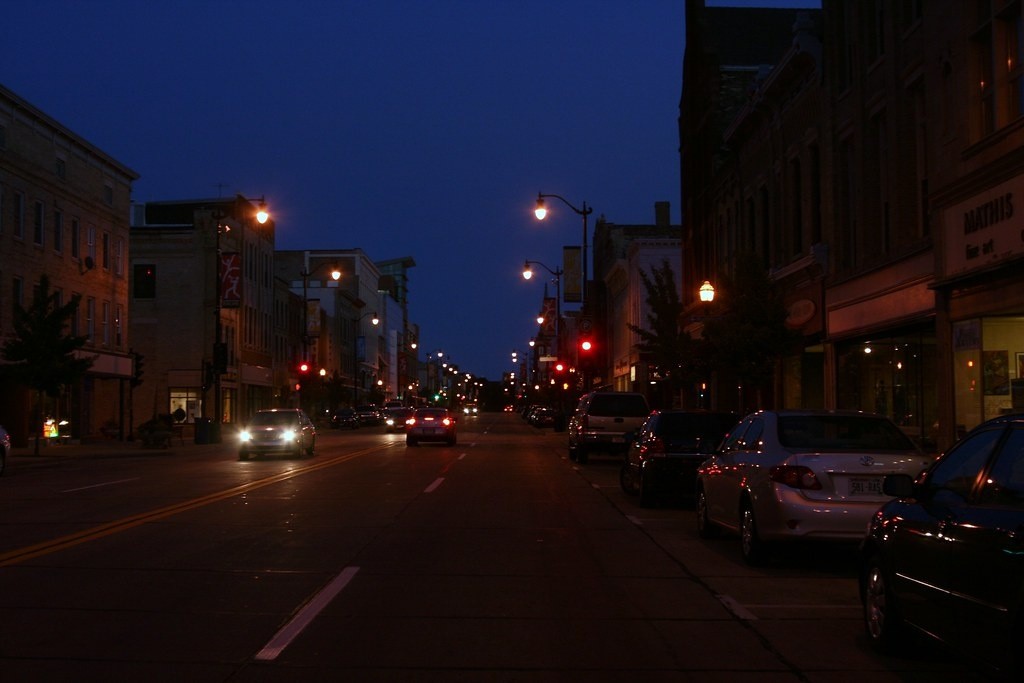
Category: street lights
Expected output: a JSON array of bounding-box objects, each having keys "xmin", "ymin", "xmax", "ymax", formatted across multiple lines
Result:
[
  {"xmin": 521, "ymin": 259, "xmax": 561, "ymax": 336},
  {"xmin": 300, "ymin": 261, "xmax": 342, "ymax": 410},
  {"xmin": 510, "ymin": 315, "xmax": 559, "ymax": 382},
  {"xmin": 532, "ymin": 190, "xmax": 594, "ymax": 314},
  {"xmin": 212, "ymin": 194, "xmax": 272, "ymax": 425},
  {"xmin": 698, "ymin": 278, "xmax": 720, "ymax": 408},
  {"xmin": 351, "ymin": 311, "xmax": 444, "ymax": 410}
]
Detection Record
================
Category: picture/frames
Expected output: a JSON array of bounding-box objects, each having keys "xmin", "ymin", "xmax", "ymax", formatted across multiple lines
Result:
[
  {"xmin": 982, "ymin": 350, "xmax": 1010, "ymax": 396},
  {"xmin": 1016, "ymin": 352, "xmax": 1024, "ymax": 379}
]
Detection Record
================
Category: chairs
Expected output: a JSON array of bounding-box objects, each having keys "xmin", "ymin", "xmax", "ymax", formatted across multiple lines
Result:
[{"xmin": 839, "ymin": 422, "xmax": 881, "ymax": 440}]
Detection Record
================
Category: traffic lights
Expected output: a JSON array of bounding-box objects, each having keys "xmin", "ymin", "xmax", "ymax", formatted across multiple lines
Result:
[
  {"xmin": 578, "ymin": 335, "xmax": 597, "ymax": 357},
  {"xmin": 135, "ymin": 353, "xmax": 144, "ymax": 379}
]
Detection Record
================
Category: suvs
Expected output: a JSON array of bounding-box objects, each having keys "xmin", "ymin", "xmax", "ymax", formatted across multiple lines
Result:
[{"xmin": 569, "ymin": 390, "xmax": 651, "ymax": 465}]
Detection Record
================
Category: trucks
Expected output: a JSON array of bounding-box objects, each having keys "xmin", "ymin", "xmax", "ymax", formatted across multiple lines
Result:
[{"xmin": 464, "ymin": 404, "xmax": 480, "ymax": 416}]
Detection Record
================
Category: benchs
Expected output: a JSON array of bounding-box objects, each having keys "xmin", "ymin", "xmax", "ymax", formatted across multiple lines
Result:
[{"xmin": 136, "ymin": 425, "xmax": 184, "ymax": 448}]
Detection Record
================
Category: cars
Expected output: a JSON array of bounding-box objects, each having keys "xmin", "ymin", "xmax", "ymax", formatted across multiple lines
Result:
[
  {"xmin": 330, "ymin": 401, "xmax": 415, "ymax": 433},
  {"xmin": 856, "ymin": 413, "xmax": 1023, "ymax": 683},
  {"xmin": 521, "ymin": 404, "xmax": 558, "ymax": 428},
  {"xmin": 695, "ymin": 407, "xmax": 936, "ymax": 555},
  {"xmin": 406, "ymin": 408, "xmax": 458, "ymax": 447},
  {"xmin": 620, "ymin": 409, "xmax": 743, "ymax": 503},
  {"xmin": 0, "ymin": 425, "xmax": 12, "ymax": 479},
  {"xmin": 239, "ymin": 406, "xmax": 316, "ymax": 462}
]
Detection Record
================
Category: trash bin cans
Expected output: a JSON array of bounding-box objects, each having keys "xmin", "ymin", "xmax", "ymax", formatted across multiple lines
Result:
[
  {"xmin": 194, "ymin": 417, "xmax": 211, "ymax": 444},
  {"xmin": 555, "ymin": 414, "xmax": 565, "ymax": 432}
]
